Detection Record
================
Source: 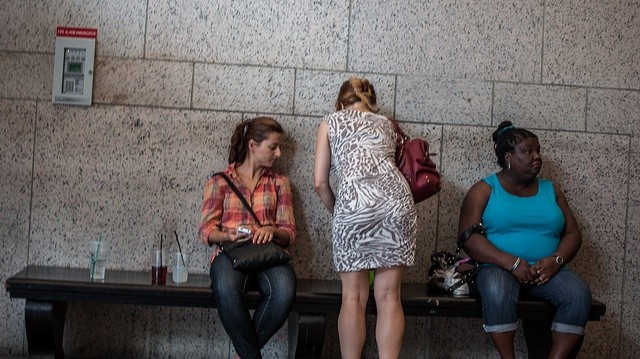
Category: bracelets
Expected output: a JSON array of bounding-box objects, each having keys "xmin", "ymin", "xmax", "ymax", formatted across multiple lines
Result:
[{"xmin": 509, "ymin": 256, "xmax": 523, "ymax": 272}]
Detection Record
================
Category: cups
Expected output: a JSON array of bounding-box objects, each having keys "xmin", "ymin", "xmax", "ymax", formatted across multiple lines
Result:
[
  {"xmin": 172, "ymin": 252, "xmax": 190, "ymax": 283},
  {"xmin": 151, "ymin": 247, "xmax": 170, "ymax": 287},
  {"xmin": 90, "ymin": 241, "xmax": 109, "ymax": 279}
]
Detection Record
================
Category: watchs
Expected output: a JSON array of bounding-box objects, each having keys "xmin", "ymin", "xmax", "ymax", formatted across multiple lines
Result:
[{"xmin": 552, "ymin": 254, "xmax": 565, "ymax": 267}]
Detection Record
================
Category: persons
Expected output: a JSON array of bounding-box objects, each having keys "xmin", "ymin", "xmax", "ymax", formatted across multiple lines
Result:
[
  {"xmin": 199, "ymin": 117, "xmax": 298, "ymax": 359},
  {"xmin": 313, "ymin": 78, "xmax": 419, "ymax": 359},
  {"xmin": 456, "ymin": 120, "xmax": 591, "ymax": 358}
]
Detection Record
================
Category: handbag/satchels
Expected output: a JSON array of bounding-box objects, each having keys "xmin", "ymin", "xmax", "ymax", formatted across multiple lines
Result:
[
  {"xmin": 220, "ymin": 239, "xmax": 292, "ymax": 268},
  {"xmin": 390, "ymin": 116, "xmax": 441, "ymax": 203},
  {"xmin": 429, "ymin": 252, "xmax": 487, "ymax": 299}
]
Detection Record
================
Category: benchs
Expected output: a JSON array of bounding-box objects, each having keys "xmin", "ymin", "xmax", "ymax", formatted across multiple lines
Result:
[{"xmin": 5, "ymin": 266, "xmax": 606, "ymax": 359}]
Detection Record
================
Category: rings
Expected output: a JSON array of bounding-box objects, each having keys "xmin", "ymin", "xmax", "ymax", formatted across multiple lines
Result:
[
  {"xmin": 537, "ymin": 270, "xmax": 542, "ymax": 274},
  {"xmin": 538, "ymin": 275, "xmax": 544, "ymax": 282}
]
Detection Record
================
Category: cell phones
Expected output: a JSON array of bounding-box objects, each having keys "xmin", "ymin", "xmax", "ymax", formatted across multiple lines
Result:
[{"xmin": 238, "ymin": 228, "xmax": 251, "ymax": 237}]
